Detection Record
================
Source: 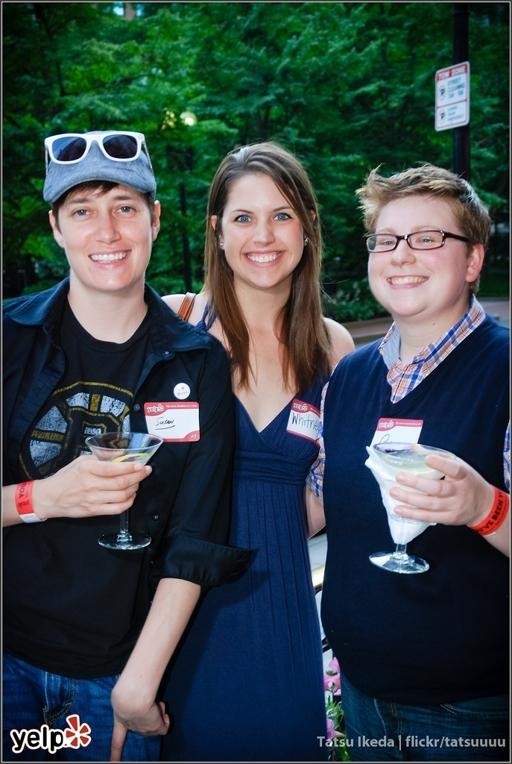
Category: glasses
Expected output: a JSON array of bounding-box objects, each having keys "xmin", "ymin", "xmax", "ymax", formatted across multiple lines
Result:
[
  {"xmin": 43, "ymin": 130, "xmax": 151, "ymax": 175},
  {"xmin": 365, "ymin": 229, "xmax": 467, "ymax": 254}
]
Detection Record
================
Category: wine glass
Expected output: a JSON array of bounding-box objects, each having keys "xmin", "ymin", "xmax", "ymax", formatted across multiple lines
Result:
[
  {"xmin": 365, "ymin": 440, "xmax": 458, "ymax": 575},
  {"xmin": 82, "ymin": 430, "xmax": 164, "ymax": 555}
]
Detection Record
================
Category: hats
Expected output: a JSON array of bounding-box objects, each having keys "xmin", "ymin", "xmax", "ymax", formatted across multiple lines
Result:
[{"xmin": 43, "ymin": 129, "xmax": 157, "ymax": 204}]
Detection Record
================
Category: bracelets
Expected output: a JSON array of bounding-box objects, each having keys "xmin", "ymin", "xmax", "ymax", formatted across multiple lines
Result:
[
  {"xmin": 467, "ymin": 487, "xmax": 508, "ymax": 540},
  {"xmin": 16, "ymin": 479, "xmax": 47, "ymax": 527}
]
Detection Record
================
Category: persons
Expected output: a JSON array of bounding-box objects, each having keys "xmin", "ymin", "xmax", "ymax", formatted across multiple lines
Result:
[
  {"xmin": 157, "ymin": 140, "xmax": 357, "ymax": 755},
  {"xmin": 305, "ymin": 165, "xmax": 509, "ymax": 758},
  {"xmin": 4, "ymin": 127, "xmax": 261, "ymax": 762}
]
